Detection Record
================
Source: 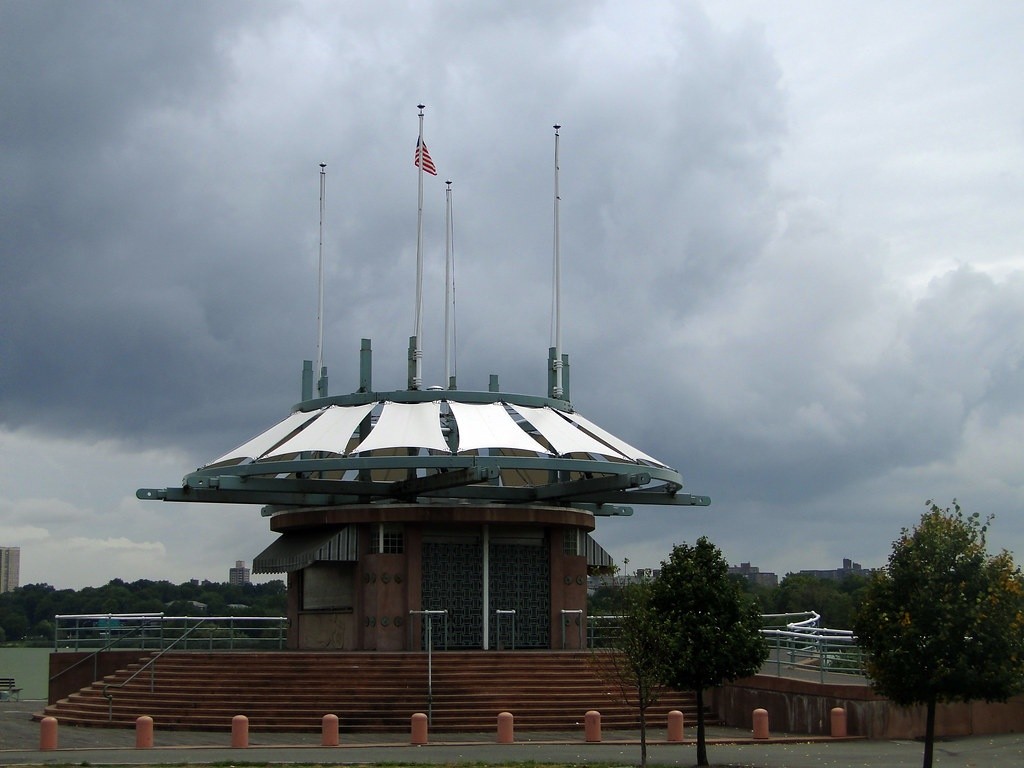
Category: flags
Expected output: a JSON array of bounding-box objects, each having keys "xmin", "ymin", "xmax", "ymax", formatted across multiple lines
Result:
[{"xmin": 415, "ymin": 135, "xmax": 437, "ymax": 177}]
